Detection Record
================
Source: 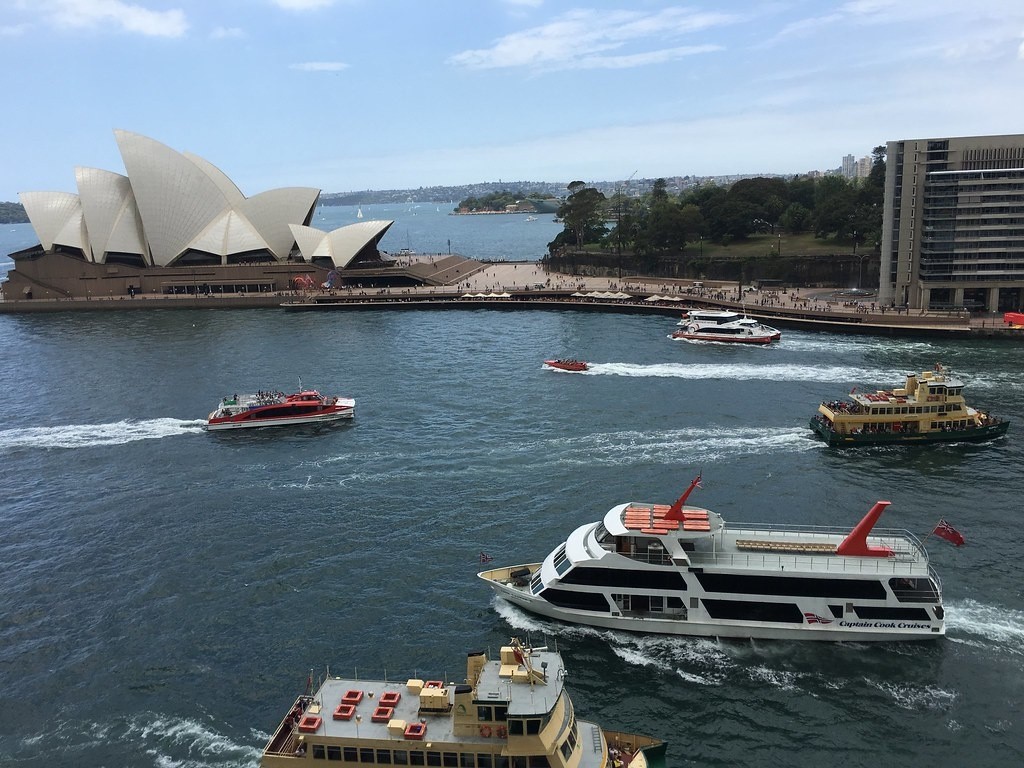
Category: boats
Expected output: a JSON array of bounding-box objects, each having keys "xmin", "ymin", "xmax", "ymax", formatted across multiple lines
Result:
[
  {"xmin": 671, "ymin": 316, "xmax": 782, "ymax": 344},
  {"xmin": 809, "ymin": 363, "xmax": 1010, "ymax": 448},
  {"xmin": 477, "ymin": 472, "xmax": 945, "ymax": 641},
  {"xmin": 203, "ymin": 375, "xmax": 356, "ymax": 430},
  {"xmin": 602, "ymin": 729, "xmax": 669, "ymax": 756},
  {"xmin": 262, "ymin": 630, "xmax": 649, "ymax": 768},
  {"xmin": 677, "ymin": 309, "xmax": 739, "ymax": 327}
]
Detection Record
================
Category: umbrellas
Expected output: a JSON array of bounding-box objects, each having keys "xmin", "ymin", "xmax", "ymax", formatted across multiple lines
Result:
[
  {"xmin": 571, "ymin": 290, "xmax": 633, "ymax": 300},
  {"xmin": 461, "ymin": 292, "xmax": 512, "ymax": 298},
  {"xmin": 644, "ymin": 295, "xmax": 684, "ymax": 303}
]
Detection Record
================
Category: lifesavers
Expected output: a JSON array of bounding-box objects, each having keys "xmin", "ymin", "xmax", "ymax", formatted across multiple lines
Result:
[
  {"xmin": 480, "ymin": 724, "xmax": 508, "ymax": 739},
  {"xmin": 927, "ymin": 395, "xmax": 940, "ymax": 402}
]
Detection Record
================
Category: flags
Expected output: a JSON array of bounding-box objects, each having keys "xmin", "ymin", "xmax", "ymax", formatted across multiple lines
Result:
[{"xmin": 932, "ymin": 519, "xmax": 964, "ymax": 547}]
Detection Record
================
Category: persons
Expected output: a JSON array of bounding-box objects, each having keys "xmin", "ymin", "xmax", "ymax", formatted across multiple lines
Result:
[
  {"xmin": 284, "ymin": 696, "xmax": 309, "ymax": 728},
  {"xmin": 850, "ymin": 425, "xmax": 916, "ymax": 435},
  {"xmin": 941, "ymin": 423, "xmax": 958, "ymax": 432},
  {"xmin": 609, "ymin": 742, "xmax": 633, "ymax": 768},
  {"xmin": 973, "ymin": 406, "xmax": 1003, "ymax": 427},
  {"xmin": 607, "ymin": 278, "xmax": 646, "ymax": 291},
  {"xmin": 814, "ymin": 414, "xmax": 836, "ymax": 433},
  {"xmin": 527, "ymin": 297, "xmax": 709, "ymax": 310},
  {"xmin": 755, "ymin": 287, "xmax": 909, "ymax": 316},
  {"xmin": 823, "ymin": 399, "xmax": 860, "ymax": 414},
  {"xmin": 554, "ymin": 357, "xmax": 577, "ymax": 366},
  {"xmin": 255, "ymin": 388, "xmax": 283, "ymax": 405},
  {"xmin": 660, "ymin": 283, "xmax": 755, "ymax": 302},
  {"xmin": 221, "ymin": 392, "xmax": 239, "ymax": 415}
]
